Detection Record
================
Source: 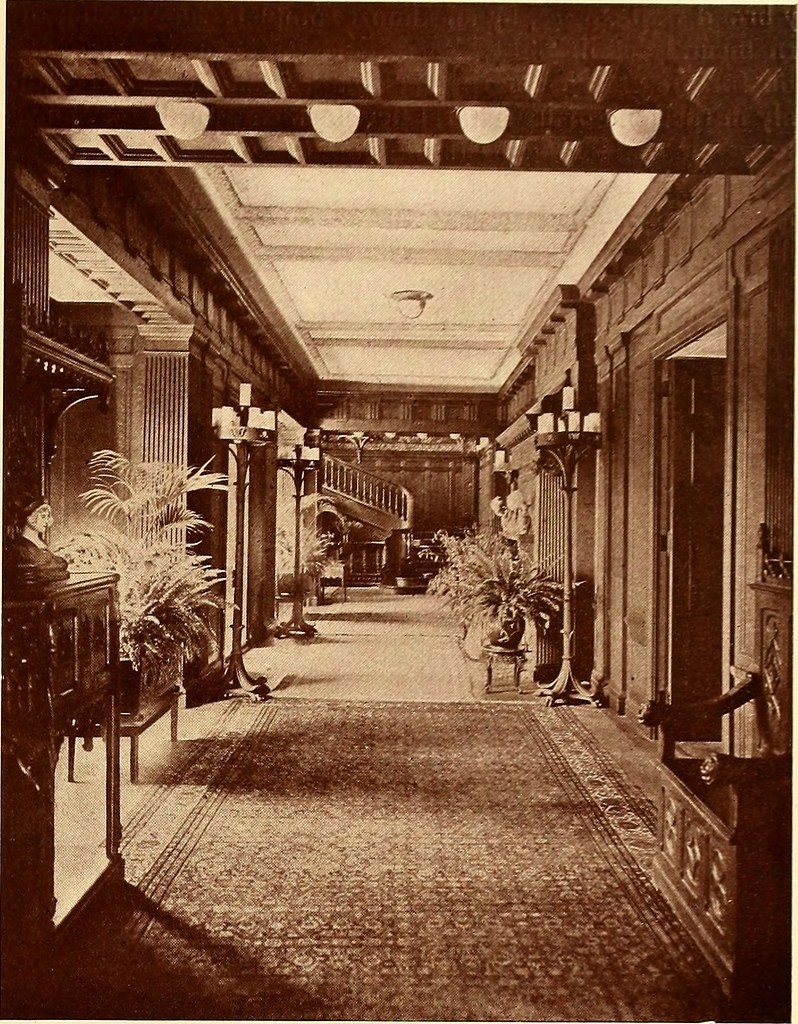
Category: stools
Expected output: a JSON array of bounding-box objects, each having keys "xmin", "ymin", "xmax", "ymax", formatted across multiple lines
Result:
[{"xmin": 483, "ymin": 643, "xmax": 529, "ymax": 694}]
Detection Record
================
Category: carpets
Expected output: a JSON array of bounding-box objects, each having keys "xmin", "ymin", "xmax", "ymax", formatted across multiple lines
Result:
[
  {"xmin": 0, "ymin": 696, "xmax": 791, "ymax": 1020},
  {"xmin": 302, "ymin": 587, "xmax": 470, "ymax": 637}
]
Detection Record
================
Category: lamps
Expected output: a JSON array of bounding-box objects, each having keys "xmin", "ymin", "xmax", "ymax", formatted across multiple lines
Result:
[
  {"xmin": 458, "ymin": 106, "xmax": 510, "ymax": 144},
  {"xmin": 307, "ymin": 103, "xmax": 361, "ymax": 143},
  {"xmin": 214, "ymin": 384, "xmax": 278, "ymax": 703},
  {"xmin": 608, "ymin": 110, "xmax": 663, "ymax": 145},
  {"xmin": 534, "ymin": 386, "xmax": 603, "ymax": 707},
  {"xmin": 274, "ymin": 435, "xmax": 320, "ymax": 638},
  {"xmin": 155, "ymin": 101, "xmax": 210, "ymax": 141},
  {"xmin": 391, "ymin": 290, "xmax": 433, "ymax": 319}
]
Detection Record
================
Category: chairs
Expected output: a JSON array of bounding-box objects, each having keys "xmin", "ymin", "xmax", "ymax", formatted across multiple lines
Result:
[
  {"xmin": 635, "ymin": 523, "xmax": 793, "ymax": 1003},
  {"xmin": 314, "ymin": 560, "xmax": 346, "ymax": 604}
]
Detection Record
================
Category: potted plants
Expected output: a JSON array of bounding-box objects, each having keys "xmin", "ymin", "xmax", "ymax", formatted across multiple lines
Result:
[
  {"xmin": 280, "ymin": 527, "xmax": 334, "ymax": 607},
  {"xmin": 395, "ymin": 567, "xmax": 422, "ymax": 587},
  {"xmin": 461, "ymin": 553, "xmax": 587, "ymax": 653},
  {"xmin": 118, "ymin": 592, "xmax": 167, "ymax": 714}
]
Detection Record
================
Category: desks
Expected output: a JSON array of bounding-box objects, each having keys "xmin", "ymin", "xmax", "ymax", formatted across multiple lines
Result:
[
  {"xmin": 68, "ymin": 687, "xmax": 182, "ymax": 783},
  {"xmin": 0, "ymin": 310, "xmax": 126, "ymax": 1020}
]
